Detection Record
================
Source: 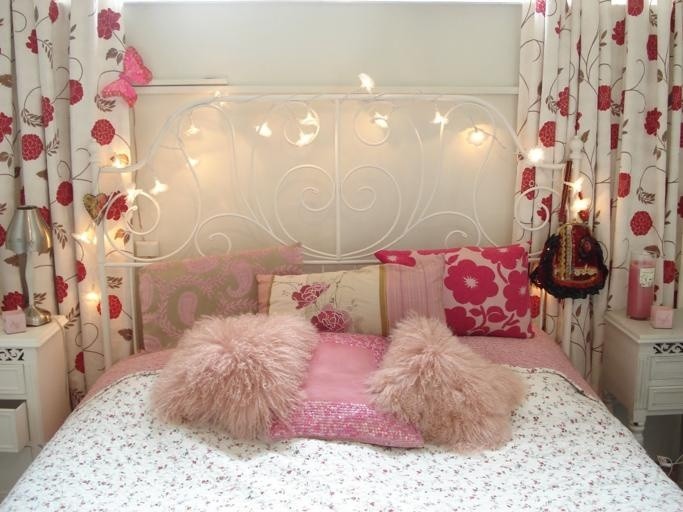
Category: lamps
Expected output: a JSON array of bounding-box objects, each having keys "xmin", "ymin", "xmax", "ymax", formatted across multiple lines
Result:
[{"xmin": 2, "ymin": 206, "xmax": 61, "ymax": 326}]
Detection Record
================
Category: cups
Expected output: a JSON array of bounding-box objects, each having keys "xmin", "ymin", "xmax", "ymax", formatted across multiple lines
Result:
[{"xmin": 627, "ymin": 248, "xmax": 656, "ymax": 318}]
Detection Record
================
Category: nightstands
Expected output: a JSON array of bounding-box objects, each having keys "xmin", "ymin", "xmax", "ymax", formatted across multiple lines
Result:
[
  {"xmin": 598, "ymin": 310, "xmax": 682, "ymax": 442},
  {"xmin": 0, "ymin": 312, "xmax": 71, "ymax": 494}
]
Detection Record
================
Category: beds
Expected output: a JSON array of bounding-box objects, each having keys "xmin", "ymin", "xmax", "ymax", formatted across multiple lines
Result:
[{"xmin": 0, "ymin": 88, "xmax": 683, "ymax": 511}]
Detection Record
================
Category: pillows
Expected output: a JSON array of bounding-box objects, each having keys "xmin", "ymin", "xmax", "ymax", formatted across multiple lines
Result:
[
  {"xmin": 361, "ymin": 310, "xmax": 519, "ymax": 449},
  {"xmin": 373, "ymin": 246, "xmax": 536, "ymax": 340},
  {"xmin": 149, "ymin": 309, "xmax": 315, "ymax": 446},
  {"xmin": 136, "ymin": 241, "xmax": 304, "ymax": 350},
  {"xmin": 274, "ymin": 330, "xmax": 419, "ymax": 448},
  {"xmin": 245, "ymin": 255, "xmax": 449, "ymax": 339}
]
